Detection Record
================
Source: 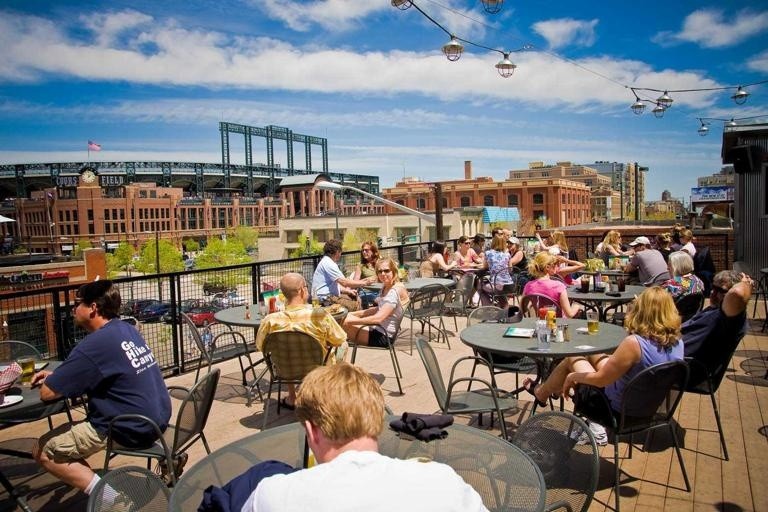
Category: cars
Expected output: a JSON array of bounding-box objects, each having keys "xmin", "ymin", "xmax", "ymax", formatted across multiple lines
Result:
[
  {"xmin": 182, "ymin": 255, "xmax": 189, "ymax": 260},
  {"xmin": 183, "ymin": 259, "xmax": 195, "ymax": 268},
  {"xmin": 250, "ymin": 265, "xmax": 269, "ymax": 276},
  {"xmin": 114, "ymin": 292, "xmax": 247, "ymax": 333},
  {"xmin": 131, "ymin": 257, "xmax": 140, "ymax": 262}
]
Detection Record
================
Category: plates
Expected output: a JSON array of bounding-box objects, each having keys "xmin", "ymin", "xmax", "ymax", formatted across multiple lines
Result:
[
  {"xmin": 0, "ymin": 395, "xmax": 24, "ymax": 407},
  {"xmin": 325, "ymin": 307, "xmax": 345, "ymax": 315},
  {"xmin": 470, "ymin": 265, "xmax": 479, "ymax": 268}
]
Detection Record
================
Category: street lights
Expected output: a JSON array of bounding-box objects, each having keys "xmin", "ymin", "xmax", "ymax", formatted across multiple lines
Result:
[
  {"xmin": 634, "ymin": 161, "xmax": 650, "ymax": 221},
  {"xmin": 313, "ymin": 179, "xmax": 445, "ymax": 254},
  {"xmin": 617, "ymin": 182, "xmax": 623, "ymax": 221}
]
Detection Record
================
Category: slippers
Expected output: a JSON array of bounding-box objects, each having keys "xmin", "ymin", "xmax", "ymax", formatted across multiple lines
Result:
[
  {"xmin": 524, "ymin": 378, "xmax": 550, "ymax": 408},
  {"xmin": 279, "ymin": 396, "xmax": 297, "ymax": 412}
]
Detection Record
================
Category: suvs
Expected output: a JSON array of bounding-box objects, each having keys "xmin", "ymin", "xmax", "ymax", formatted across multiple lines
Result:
[{"xmin": 203, "ymin": 280, "xmax": 238, "ymax": 296}]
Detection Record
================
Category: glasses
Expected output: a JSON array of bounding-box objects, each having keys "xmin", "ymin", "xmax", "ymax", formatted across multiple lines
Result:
[
  {"xmin": 376, "ymin": 269, "xmax": 395, "ymax": 274},
  {"xmin": 73, "ymin": 295, "xmax": 105, "ymax": 305},
  {"xmin": 295, "ymin": 283, "xmax": 309, "ymax": 293},
  {"xmin": 361, "ymin": 248, "xmax": 375, "ymax": 253},
  {"xmin": 495, "ymin": 232, "xmax": 505, "ymax": 235},
  {"xmin": 460, "ymin": 242, "xmax": 471, "ymax": 245},
  {"xmin": 711, "ymin": 283, "xmax": 742, "ymax": 295}
]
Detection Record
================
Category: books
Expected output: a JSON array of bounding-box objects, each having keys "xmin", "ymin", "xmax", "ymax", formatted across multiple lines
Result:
[{"xmin": 504, "ymin": 327, "xmax": 536, "ymax": 339}]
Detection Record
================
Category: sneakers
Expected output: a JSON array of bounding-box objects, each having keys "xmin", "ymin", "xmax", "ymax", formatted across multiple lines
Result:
[
  {"xmin": 96, "ymin": 489, "xmax": 138, "ymax": 512},
  {"xmin": 560, "ymin": 428, "xmax": 608, "ymax": 446},
  {"xmin": 157, "ymin": 446, "xmax": 190, "ymax": 487}
]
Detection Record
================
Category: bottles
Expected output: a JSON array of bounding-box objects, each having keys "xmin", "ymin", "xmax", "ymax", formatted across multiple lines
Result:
[
  {"xmin": 244, "ymin": 304, "xmax": 251, "ymax": 320},
  {"xmin": 555, "ymin": 324, "xmax": 564, "ymax": 343},
  {"xmin": 312, "ymin": 291, "xmax": 319, "ymax": 308},
  {"xmin": 562, "ymin": 323, "xmax": 571, "ymax": 341}
]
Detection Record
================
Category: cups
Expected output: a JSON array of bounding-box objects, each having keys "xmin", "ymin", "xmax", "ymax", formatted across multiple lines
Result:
[
  {"xmin": 612, "ymin": 258, "xmax": 620, "ymax": 269},
  {"xmin": 17, "ymin": 358, "xmax": 36, "ymax": 385},
  {"xmin": 586, "ymin": 311, "xmax": 600, "ymax": 336},
  {"xmin": 535, "ymin": 303, "xmax": 557, "ymax": 352},
  {"xmin": 259, "ymin": 294, "xmax": 286, "ymax": 316},
  {"xmin": 581, "ymin": 269, "xmax": 625, "ymax": 292},
  {"xmin": 398, "ymin": 265, "xmax": 416, "ymax": 282},
  {"xmin": 448, "ymin": 258, "xmax": 452, "ymax": 265}
]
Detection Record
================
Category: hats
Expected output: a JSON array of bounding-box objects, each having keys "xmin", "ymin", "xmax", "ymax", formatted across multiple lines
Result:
[{"xmin": 628, "ymin": 235, "xmax": 651, "ymax": 247}]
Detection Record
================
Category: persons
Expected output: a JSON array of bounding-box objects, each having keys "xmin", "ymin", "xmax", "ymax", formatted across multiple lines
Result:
[
  {"xmin": 29, "ymin": 279, "xmax": 173, "ymax": 511},
  {"xmin": 349, "ymin": 240, "xmax": 383, "ymax": 310},
  {"xmin": 255, "ymin": 273, "xmax": 348, "ymax": 410},
  {"xmin": 420, "ymin": 226, "xmax": 586, "ymax": 323},
  {"xmin": 310, "ymin": 238, "xmax": 376, "ymax": 313},
  {"xmin": 523, "ymin": 270, "xmax": 753, "ymax": 447},
  {"xmin": 594, "ymin": 227, "xmax": 704, "ymax": 299},
  {"xmin": 239, "ymin": 361, "xmax": 491, "ymax": 511},
  {"xmin": 342, "ymin": 256, "xmax": 411, "ymax": 348}
]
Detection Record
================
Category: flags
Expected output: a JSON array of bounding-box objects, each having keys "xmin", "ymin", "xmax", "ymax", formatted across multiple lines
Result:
[{"xmin": 87, "ymin": 139, "xmax": 102, "ymax": 152}]
